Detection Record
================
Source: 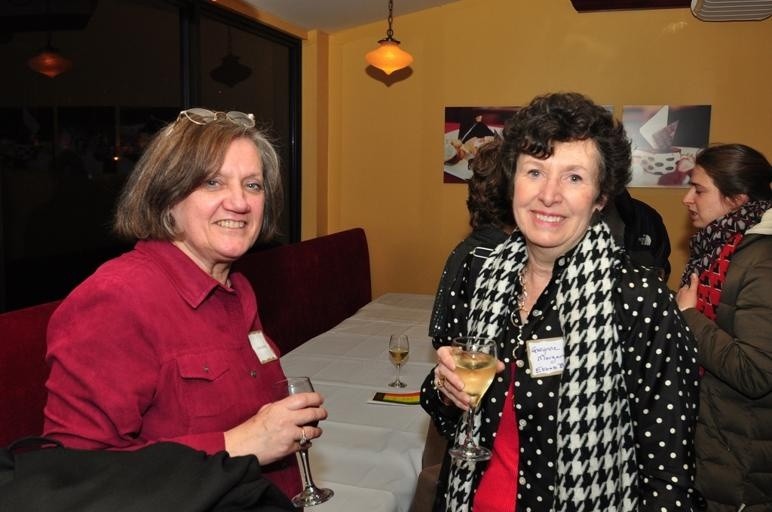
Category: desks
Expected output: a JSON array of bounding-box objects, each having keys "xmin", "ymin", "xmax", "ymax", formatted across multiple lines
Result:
[{"xmin": 274, "ymin": 292, "xmax": 437, "ymax": 512}]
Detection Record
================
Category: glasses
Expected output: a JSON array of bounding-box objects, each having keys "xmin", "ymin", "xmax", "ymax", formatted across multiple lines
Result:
[{"xmin": 178, "ymin": 108, "xmax": 256, "ymax": 128}]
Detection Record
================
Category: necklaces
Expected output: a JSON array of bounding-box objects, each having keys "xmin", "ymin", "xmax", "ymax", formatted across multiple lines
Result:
[{"xmin": 511, "ymin": 267, "xmax": 529, "ymax": 330}]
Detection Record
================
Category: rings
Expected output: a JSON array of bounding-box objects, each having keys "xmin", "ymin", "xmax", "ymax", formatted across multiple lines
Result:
[
  {"xmin": 437, "ymin": 376, "xmax": 446, "ymax": 388},
  {"xmin": 300, "ymin": 429, "xmax": 307, "ymax": 445}
]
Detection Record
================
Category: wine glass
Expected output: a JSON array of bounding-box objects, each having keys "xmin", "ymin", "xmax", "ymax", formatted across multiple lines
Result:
[
  {"xmin": 389, "ymin": 333, "xmax": 409, "ymax": 390},
  {"xmin": 444, "ymin": 338, "xmax": 497, "ymax": 464},
  {"xmin": 270, "ymin": 377, "xmax": 333, "ymax": 508}
]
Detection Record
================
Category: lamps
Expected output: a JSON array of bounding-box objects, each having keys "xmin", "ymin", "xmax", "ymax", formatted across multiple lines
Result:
[
  {"xmin": 28, "ymin": 33, "xmax": 70, "ymax": 79},
  {"xmin": 365, "ymin": 1, "xmax": 414, "ymax": 78}
]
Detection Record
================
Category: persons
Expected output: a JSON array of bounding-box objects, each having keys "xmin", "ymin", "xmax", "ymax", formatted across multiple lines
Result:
[
  {"xmin": 596, "ymin": 187, "xmax": 671, "ymax": 284},
  {"xmin": 419, "ymin": 91, "xmax": 706, "ymax": 511},
  {"xmin": 42, "ymin": 108, "xmax": 327, "ymax": 512},
  {"xmin": 430, "ymin": 140, "xmax": 517, "ymax": 346},
  {"xmin": 674, "ymin": 144, "xmax": 772, "ymax": 512}
]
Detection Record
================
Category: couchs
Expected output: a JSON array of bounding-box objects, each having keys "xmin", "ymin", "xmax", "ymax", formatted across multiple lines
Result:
[{"xmin": 1, "ymin": 229, "xmax": 371, "ymax": 461}]
